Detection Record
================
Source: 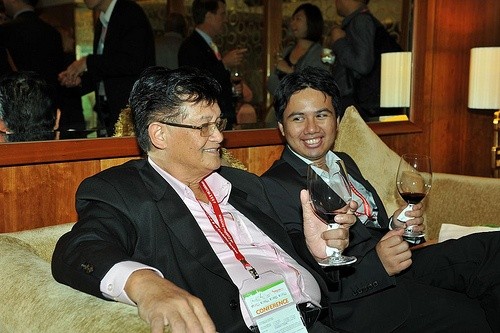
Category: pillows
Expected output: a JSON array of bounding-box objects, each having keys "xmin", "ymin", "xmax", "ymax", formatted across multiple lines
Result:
[{"xmin": 330, "ymin": 106, "xmax": 400, "ymax": 218}]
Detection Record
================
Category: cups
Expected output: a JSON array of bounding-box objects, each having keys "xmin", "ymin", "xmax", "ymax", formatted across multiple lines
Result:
[
  {"xmin": 320, "ymin": 32, "xmax": 336, "ymax": 64},
  {"xmin": 273, "ymin": 41, "xmax": 287, "ymax": 60}
]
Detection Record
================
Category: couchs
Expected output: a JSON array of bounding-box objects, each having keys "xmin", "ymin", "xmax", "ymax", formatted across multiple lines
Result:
[{"xmin": 0, "ymin": 173, "xmax": 499, "ymax": 333}]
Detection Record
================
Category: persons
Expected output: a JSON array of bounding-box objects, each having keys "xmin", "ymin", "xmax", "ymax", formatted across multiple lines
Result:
[
  {"xmin": 178, "ymin": 0, "xmax": 248, "ymax": 131},
  {"xmin": 259, "ymin": 66, "xmax": 500, "ymax": 333},
  {"xmin": 266, "ymin": 3, "xmax": 333, "ymax": 94},
  {"xmin": 58, "ymin": 0, "xmax": 156, "ymax": 138},
  {"xmin": 4, "ymin": 0, "xmax": 87, "ymax": 140},
  {"xmin": 0, "ymin": 69, "xmax": 61, "ymax": 143},
  {"xmin": 331, "ymin": 0, "xmax": 401, "ymax": 123},
  {"xmin": 51, "ymin": 67, "xmax": 358, "ymax": 333}
]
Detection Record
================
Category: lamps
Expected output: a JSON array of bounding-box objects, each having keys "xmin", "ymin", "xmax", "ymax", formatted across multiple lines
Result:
[
  {"xmin": 468, "ymin": 47, "xmax": 500, "ymax": 179},
  {"xmin": 379, "ymin": 52, "xmax": 411, "ymax": 118}
]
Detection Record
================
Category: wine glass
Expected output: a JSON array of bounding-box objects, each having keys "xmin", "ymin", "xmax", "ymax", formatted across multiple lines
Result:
[
  {"xmin": 396, "ymin": 154, "xmax": 431, "ymax": 237},
  {"xmin": 230, "ymin": 66, "xmax": 243, "ymax": 98},
  {"xmin": 307, "ymin": 160, "xmax": 357, "ymax": 267}
]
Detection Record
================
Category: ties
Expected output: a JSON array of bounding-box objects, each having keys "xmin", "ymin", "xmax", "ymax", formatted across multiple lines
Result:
[{"xmin": 211, "ymin": 41, "xmax": 222, "ymax": 61}]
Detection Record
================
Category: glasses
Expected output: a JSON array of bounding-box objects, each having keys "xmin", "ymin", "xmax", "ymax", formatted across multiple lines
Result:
[{"xmin": 158, "ymin": 120, "xmax": 226, "ymax": 137}]
